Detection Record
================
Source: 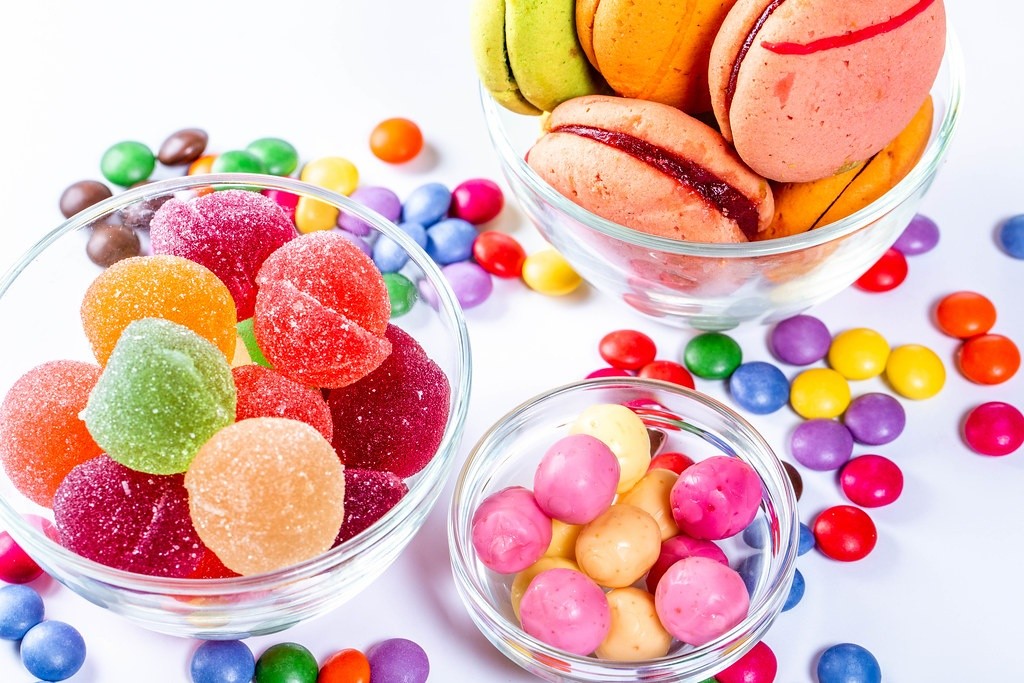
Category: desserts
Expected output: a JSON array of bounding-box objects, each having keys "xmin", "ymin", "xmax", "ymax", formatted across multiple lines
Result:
[{"xmin": 472, "ymin": 0, "xmax": 947, "ymax": 297}]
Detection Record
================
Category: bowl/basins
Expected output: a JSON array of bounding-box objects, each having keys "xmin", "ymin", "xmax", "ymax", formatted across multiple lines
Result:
[
  {"xmin": 0, "ymin": 173, "xmax": 475, "ymax": 641},
  {"xmin": 477, "ymin": 3, "xmax": 961, "ymax": 331},
  {"xmin": 447, "ymin": 375, "xmax": 802, "ymax": 683}
]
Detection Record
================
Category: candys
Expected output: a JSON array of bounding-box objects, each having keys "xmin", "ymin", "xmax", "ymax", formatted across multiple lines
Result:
[{"xmin": 0, "ymin": 118, "xmax": 1024, "ymax": 683}]
